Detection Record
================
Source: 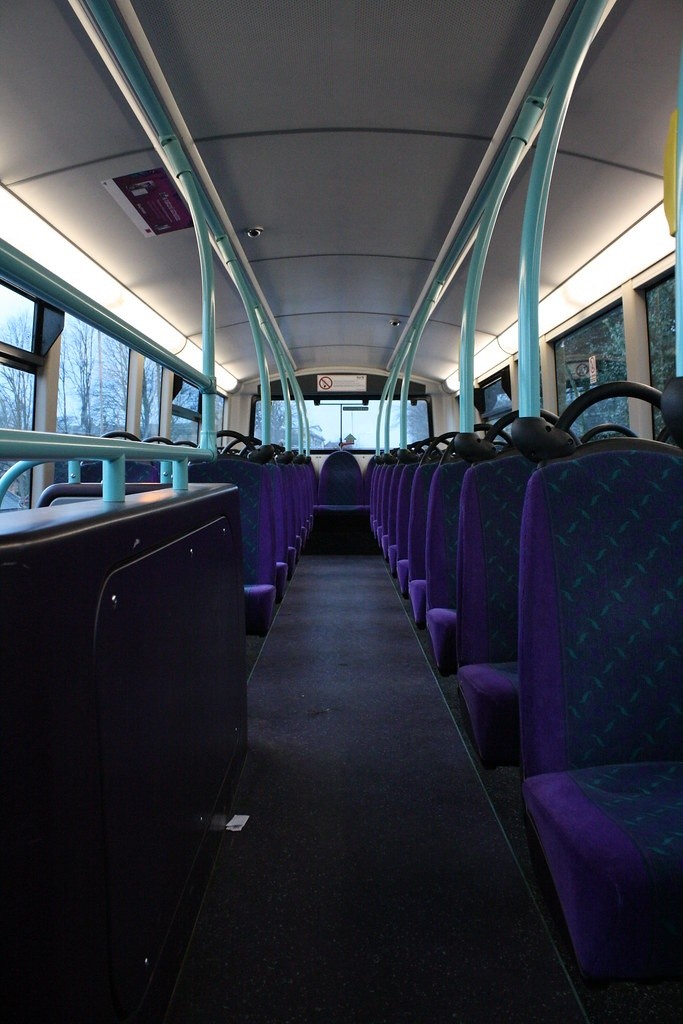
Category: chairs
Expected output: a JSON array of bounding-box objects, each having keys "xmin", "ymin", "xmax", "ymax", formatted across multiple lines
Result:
[{"xmin": 82, "ymin": 374, "xmax": 683, "ymax": 1024}]
